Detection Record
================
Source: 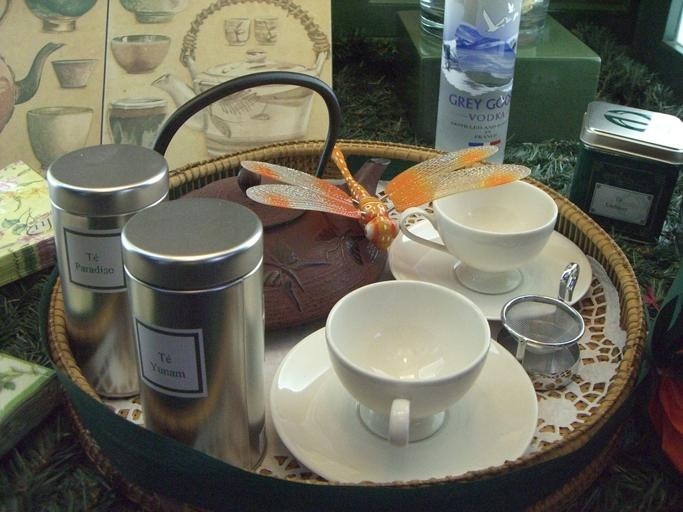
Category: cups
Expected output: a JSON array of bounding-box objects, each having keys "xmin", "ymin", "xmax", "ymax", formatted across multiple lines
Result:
[
  {"xmin": 51, "ymin": 58, "xmax": 99, "ymax": 90},
  {"xmin": 518, "ymin": 1, "xmax": 549, "ymax": 34},
  {"xmin": 417, "ymin": 1, "xmax": 442, "ymax": 47},
  {"xmin": 107, "ymin": 99, "xmax": 167, "ymax": 150},
  {"xmin": 434, "ymin": 0, "xmax": 521, "ymax": 169},
  {"xmin": 326, "ymin": 279, "xmax": 493, "ymax": 444},
  {"xmin": 500, "ymin": 262, "xmax": 587, "ymax": 391},
  {"xmin": 398, "ymin": 176, "xmax": 560, "ymax": 295},
  {"xmin": 23, "ymin": 106, "xmax": 94, "ymax": 172}
]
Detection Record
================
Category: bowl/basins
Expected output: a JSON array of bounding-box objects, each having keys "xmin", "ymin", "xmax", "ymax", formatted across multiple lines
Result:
[
  {"xmin": 109, "ymin": 35, "xmax": 171, "ymax": 74},
  {"xmin": 22, "ymin": 1, "xmax": 98, "ymax": 33},
  {"xmin": 119, "ymin": 0, "xmax": 186, "ymax": 24}
]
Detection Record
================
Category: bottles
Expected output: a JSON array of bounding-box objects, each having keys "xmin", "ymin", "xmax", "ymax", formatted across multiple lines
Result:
[
  {"xmin": 119, "ymin": 200, "xmax": 267, "ymax": 476},
  {"xmin": 45, "ymin": 144, "xmax": 171, "ymax": 398}
]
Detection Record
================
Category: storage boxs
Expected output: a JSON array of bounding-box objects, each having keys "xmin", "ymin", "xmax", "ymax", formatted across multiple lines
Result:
[{"xmin": 390, "ymin": 5, "xmax": 602, "ymax": 144}]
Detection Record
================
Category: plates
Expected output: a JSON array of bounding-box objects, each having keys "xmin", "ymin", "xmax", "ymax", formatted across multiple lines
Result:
[
  {"xmin": 386, "ymin": 216, "xmax": 592, "ymax": 321},
  {"xmin": 268, "ymin": 322, "xmax": 538, "ymax": 481}
]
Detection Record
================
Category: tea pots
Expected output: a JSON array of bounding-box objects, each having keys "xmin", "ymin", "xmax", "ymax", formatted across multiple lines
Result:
[
  {"xmin": 148, "ymin": 70, "xmax": 391, "ymax": 333},
  {"xmin": 151, "ymin": 0, "xmax": 329, "ymax": 149},
  {"xmin": 0, "ymin": 42, "xmax": 64, "ymax": 132}
]
